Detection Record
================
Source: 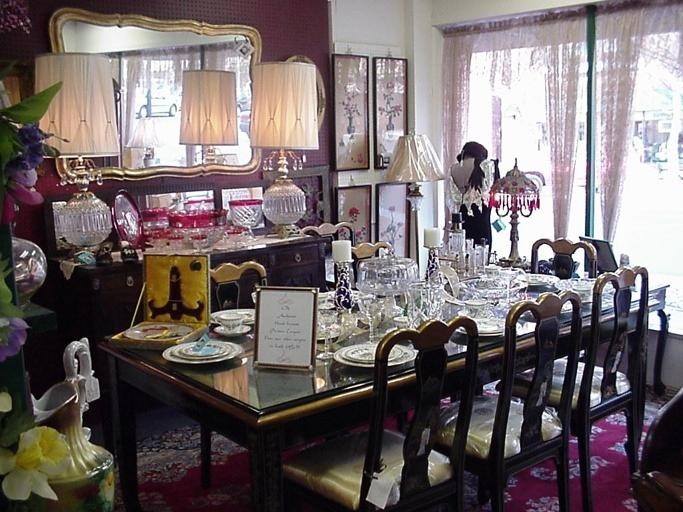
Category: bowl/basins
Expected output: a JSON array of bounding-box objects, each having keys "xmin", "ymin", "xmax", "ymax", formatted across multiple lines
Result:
[
  {"xmin": 180, "ymin": 230, "xmax": 227, "ymax": 245},
  {"xmin": 394, "ymin": 316, "xmax": 408, "ymax": 329}
]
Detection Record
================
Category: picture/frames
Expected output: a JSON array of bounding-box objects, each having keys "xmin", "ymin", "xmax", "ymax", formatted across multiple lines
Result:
[
  {"xmin": 334, "ymin": 53, "xmax": 370, "ymax": 172},
  {"xmin": 334, "ymin": 185, "xmax": 372, "ymax": 246},
  {"xmin": 376, "ymin": 181, "xmax": 410, "ymax": 261},
  {"xmin": 374, "ymin": 56, "xmax": 414, "ymax": 169}
]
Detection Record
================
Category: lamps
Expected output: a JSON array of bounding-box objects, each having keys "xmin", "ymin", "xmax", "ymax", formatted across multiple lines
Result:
[
  {"xmin": 180, "ymin": 69, "xmax": 240, "ymax": 167},
  {"xmin": 33, "ymin": 54, "xmax": 123, "ymax": 265},
  {"xmin": 386, "ymin": 135, "xmax": 446, "ymax": 212},
  {"xmin": 489, "ymin": 155, "xmax": 535, "ymax": 264},
  {"xmin": 126, "ymin": 119, "xmax": 158, "ymax": 164},
  {"xmin": 251, "ymin": 61, "xmax": 315, "ymax": 238}
]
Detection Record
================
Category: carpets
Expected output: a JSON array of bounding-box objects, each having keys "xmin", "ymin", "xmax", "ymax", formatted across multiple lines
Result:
[{"xmin": 75, "ymin": 384, "xmax": 683, "ymax": 512}]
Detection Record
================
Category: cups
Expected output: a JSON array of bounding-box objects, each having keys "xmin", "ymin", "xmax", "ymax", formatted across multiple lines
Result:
[
  {"xmin": 358, "ymin": 289, "xmax": 386, "ymax": 343},
  {"xmin": 318, "ymin": 308, "xmax": 336, "ymax": 360},
  {"xmin": 192, "ymin": 235, "xmax": 208, "ymax": 253},
  {"xmin": 184, "ymin": 197, "xmax": 214, "ymax": 212},
  {"xmin": 216, "ymin": 311, "xmax": 244, "ymax": 332},
  {"xmin": 229, "ymin": 199, "xmax": 263, "ymax": 242},
  {"xmin": 227, "ymin": 230, "xmax": 243, "ymax": 249},
  {"xmin": 465, "ymin": 300, "xmax": 487, "ymax": 319}
]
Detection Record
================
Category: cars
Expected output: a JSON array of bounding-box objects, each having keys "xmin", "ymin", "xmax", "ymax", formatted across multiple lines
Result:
[{"xmin": 131, "ymin": 91, "xmax": 176, "ymax": 118}]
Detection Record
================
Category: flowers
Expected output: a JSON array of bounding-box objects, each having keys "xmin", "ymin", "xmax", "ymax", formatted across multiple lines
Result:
[{"xmin": 0, "ymin": 77, "xmax": 77, "ymax": 503}]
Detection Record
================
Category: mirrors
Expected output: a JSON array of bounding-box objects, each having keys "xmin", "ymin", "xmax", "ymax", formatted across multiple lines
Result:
[{"xmin": 49, "ymin": 7, "xmax": 263, "ymax": 179}]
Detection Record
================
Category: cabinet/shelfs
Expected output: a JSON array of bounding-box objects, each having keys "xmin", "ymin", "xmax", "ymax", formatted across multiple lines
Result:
[{"xmin": 50, "ymin": 230, "xmax": 332, "ymax": 420}]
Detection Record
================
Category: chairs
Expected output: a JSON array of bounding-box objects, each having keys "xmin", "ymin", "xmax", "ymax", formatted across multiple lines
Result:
[
  {"xmin": 282, "ymin": 318, "xmax": 479, "ymax": 511},
  {"xmin": 496, "ymin": 267, "xmax": 650, "ymax": 511},
  {"xmin": 417, "ymin": 292, "xmax": 584, "ymax": 511},
  {"xmin": 325, "ymin": 243, "xmax": 394, "ymax": 288},
  {"xmin": 531, "ymin": 237, "xmax": 599, "ymax": 361},
  {"xmin": 300, "ymin": 223, "xmax": 361, "ymax": 287},
  {"xmin": 202, "ymin": 260, "xmax": 266, "ymax": 485},
  {"xmin": 631, "ymin": 384, "xmax": 681, "ymax": 511}
]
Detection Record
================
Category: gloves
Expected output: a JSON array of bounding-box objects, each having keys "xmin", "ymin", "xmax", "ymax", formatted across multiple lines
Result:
[{"xmin": 468, "ymin": 167, "xmax": 487, "ymax": 190}]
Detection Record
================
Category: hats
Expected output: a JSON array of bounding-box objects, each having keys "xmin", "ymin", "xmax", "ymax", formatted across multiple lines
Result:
[{"xmin": 457, "ymin": 143, "xmax": 488, "ymax": 162}]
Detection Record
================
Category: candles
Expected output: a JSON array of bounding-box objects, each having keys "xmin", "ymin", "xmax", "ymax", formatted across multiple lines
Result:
[
  {"xmin": 423, "ymin": 228, "xmax": 444, "ymax": 245},
  {"xmin": 331, "ymin": 241, "xmax": 352, "ymax": 263}
]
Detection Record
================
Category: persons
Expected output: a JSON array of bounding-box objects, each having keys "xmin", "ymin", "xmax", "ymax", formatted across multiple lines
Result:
[{"xmin": 447, "ymin": 140, "xmax": 492, "ymax": 265}]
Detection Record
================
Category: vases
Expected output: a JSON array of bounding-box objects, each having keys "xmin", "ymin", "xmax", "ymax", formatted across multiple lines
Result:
[{"xmin": 0, "ymin": 336, "xmax": 118, "ymax": 512}]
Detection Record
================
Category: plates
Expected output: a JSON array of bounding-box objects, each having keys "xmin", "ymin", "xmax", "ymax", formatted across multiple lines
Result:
[
  {"xmin": 512, "ymin": 274, "xmax": 560, "ymax": 287},
  {"xmin": 350, "ymin": 347, "xmax": 396, "ymax": 361},
  {"xmin": 183, "ymin": 344, "xmax": 226, "ymax": 356},
  {"xmin": 171, "ymin": 342, "xmax": 232, "ymax": 360},
  {"xmin": 125, "ymin": 324, "xmax": 192, "ymax": 340},
  {"xmin": 460, "ymin": 320, "xmax": 505, "ymax": 334},
  {"xmin": 333, "ymin": 344, "xmax": 417, "ymax": 368},
  {"xmin": 209, "ymin": 309, "xmax": 256, "ymax": 326},
  {"xmin": 341, "ymin": 345, "xmax": 404, "ymax": 363},
  {"xmin": 554, "ymin": 280, "xmax": 614, "ymax": 296},
  {"xmin": 315, "ymin": 324, "xmax": 341, "ymax": 340},
  {"xmin": 214, "ymin": 325, "xmax": 251, "ymax": 337},
  {"xmin": 455, "ymin": 319, "xmax": 522, "ymax": 336},
  {"xmin": 162, "ymin": 341, "xmax": 243, "ymax": 364},
  {"xmin": 239, "ymin": 311, "xmax": 253, "ymax": 321}
]
